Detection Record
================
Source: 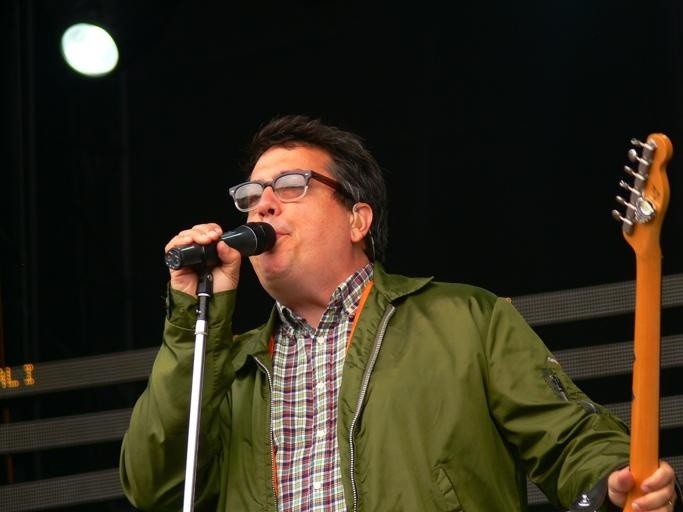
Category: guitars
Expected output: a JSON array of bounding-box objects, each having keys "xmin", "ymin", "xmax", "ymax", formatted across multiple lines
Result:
[{"xmin": 577, "ymin": 131, "xmax": 682, "ymax": 508}]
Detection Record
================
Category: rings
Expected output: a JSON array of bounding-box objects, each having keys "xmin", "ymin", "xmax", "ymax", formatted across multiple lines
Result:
[{"xmin": 668, "ymin": 500, "xmax": 673, "ymax": 509}]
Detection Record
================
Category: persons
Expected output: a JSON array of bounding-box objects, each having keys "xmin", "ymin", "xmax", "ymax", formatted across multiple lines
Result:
[{"xmin": 118, "ymin": 113, "xmax": 683, "ymax": 512}]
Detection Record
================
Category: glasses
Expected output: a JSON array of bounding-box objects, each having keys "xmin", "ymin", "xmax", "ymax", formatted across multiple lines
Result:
[{"xmin": 229, "ymin": 169, "xmax": 355, "ymax": 213}]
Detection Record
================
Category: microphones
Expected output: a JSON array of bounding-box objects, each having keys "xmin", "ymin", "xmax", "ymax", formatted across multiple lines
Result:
[{"xmin": 165, "ymin": 222, "xmax": 276, "ymax": 269}]
